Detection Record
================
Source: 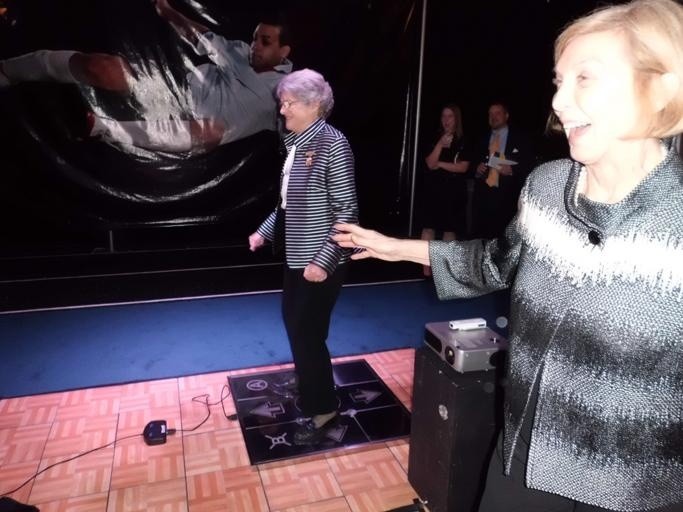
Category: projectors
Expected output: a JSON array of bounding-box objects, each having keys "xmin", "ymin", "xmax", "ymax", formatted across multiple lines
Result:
[{"xmin": 422, "ymin": 318, "xmax": 505, "ymax": 375}]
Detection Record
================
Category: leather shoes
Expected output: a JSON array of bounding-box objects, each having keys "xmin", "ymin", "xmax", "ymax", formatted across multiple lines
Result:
[{"xmin": 288, "ymin": 409, "xmax": 342, "ymax": 445}]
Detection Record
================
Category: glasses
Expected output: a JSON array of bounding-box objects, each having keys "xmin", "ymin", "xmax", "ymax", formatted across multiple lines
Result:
[{"xmin": 277, "ymin": 98, "xmax": 311, "ymax": 106}]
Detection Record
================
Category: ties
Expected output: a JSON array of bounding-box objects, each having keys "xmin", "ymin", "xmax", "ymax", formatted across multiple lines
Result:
[{"xmin": 485, "ymin": 133, "xmax": 500, "ymax": 188}]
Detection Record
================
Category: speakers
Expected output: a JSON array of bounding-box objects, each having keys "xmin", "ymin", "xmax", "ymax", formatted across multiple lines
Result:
[{"xmin": 405, "ymin": 344, "xmax": 506, "ymax": 512}]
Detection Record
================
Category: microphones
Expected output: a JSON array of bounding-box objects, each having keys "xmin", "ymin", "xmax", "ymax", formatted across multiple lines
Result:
[{"xmin": 485, "ymin": 151, "xmax": 500, "ymax": 165}]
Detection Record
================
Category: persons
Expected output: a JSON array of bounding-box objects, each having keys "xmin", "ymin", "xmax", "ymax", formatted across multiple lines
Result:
[
  {"xmin": 247, "ymin": 67, "xmax": 368, "ymax": 447},
  {"xmin": 413, "ymin": 100, "xmax": 470, "ymax": 282},
  {"xmin": 330, "ymin": 0, "xmax": 682, "ymax": 512},
  {"xmin": 1, "ymin": 0, "xmax": 304, "ymax": 158},
  {"xmin": 470, "ymin": 95, "xmax": 538, "ymax": 247}
]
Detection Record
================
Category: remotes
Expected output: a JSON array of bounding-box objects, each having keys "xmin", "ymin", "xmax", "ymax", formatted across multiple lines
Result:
[{"xmin": 450, "ymin": 315, "xmax": 485, "ymax": 332}]
